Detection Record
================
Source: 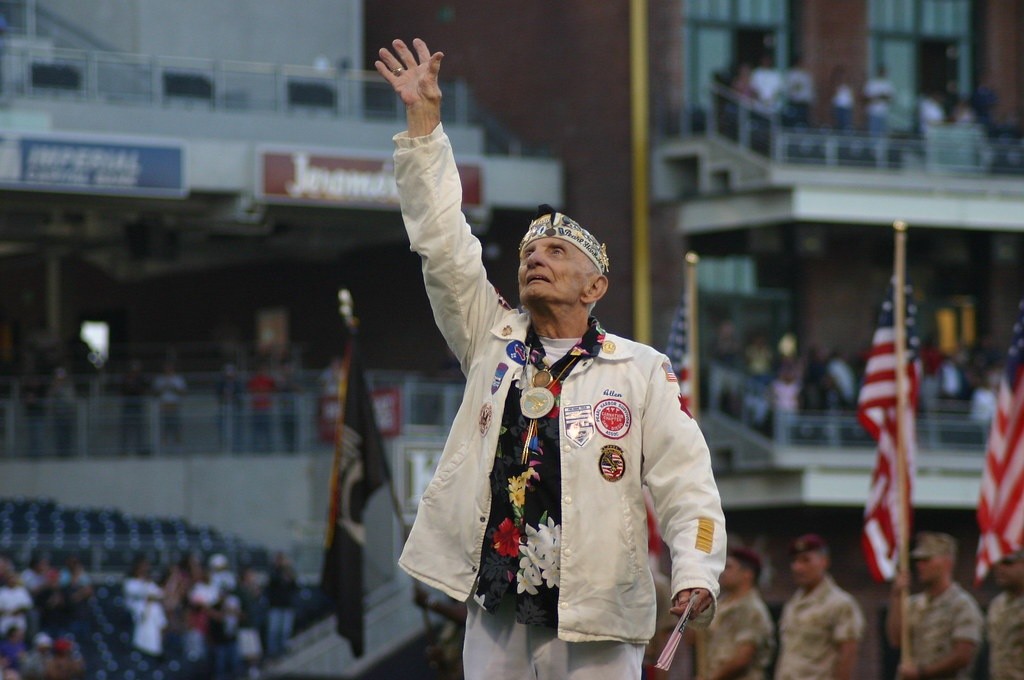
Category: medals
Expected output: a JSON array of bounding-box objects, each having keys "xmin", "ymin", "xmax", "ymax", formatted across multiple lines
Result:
[
  {"xmin": 520, "ymin": 385, "xmax": 554, "ymax": 419},
  {"xmin": 534, "ymin": 369, "xmax": 554, "ymax": 388}
]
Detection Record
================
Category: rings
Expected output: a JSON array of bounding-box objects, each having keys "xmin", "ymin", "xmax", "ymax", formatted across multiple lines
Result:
[{"xmin": 393, "ymin": 67, "xmax": 404, "ymax": 75}]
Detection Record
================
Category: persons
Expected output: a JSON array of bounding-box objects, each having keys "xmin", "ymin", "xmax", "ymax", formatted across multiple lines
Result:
[
  {"xmin": 374, "ymin": 37, "xmax": 727, "ymax": 680},
  {"xmin": 982, "ymin": 549, "xmax": 1024, "ymax": 679},
  {"xmin": 733, "ymin": 322, "xmax": 1007, "ymax": 446},
  {"xmin": 692, "ymin": 547, "xmax": 778, "ymax": 680},
  {"xmin": 0, "ymin": 350, "xmax": 340, "ymax": 460},
  {"xmin": 887, "ymin": 532, "xmax": 983, "ymax": 680},
  {"xmin": 0, "ymin": 546, "xmax": 300, "ymax": 680},
  {"xmin": 773, "ymin": 535, "xmax": 863, "ymax": 680},
  {"xmin": 412, "ymin": 591, "xmax": 467, "ymax": 680},
  {"xmin": 705, "ymin": 46, "xmax": 997, "ymax": 167}
]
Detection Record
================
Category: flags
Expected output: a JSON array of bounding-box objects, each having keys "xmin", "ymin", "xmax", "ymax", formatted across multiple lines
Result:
[
  {"xmin": 309, "ymin": 328, "xmax": 391, "ymax": 658},
  {"xmin": 971, "ymin": 296, "xmax": 1024, "ymax": 585},
  {"xmin": 640, "ymin": 291, "xmax": 691, "ymax": 558},
  {"xmin": 653, "ymin": 595, "xmax": 698, "ymax": 671},
  {"xmin": 853, "ymin": 260, "xmax": 924, "ymax": 582}
]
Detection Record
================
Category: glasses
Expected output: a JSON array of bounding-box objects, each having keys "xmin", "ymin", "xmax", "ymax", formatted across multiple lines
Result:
[
  {"xmin": 913, "ymin": 555, "xmax": 931, "ymax": 562},
  {"xmin": 1001, "ymin": 553, "xmax": 1022, "ymax": 564}
]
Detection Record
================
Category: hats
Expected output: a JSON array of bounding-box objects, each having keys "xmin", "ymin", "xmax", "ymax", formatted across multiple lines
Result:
[
  {"xmin": 910, "ymin": 533, "xmax": 959, "ymax": 560},
  {"xmin": 786, "ymin": 533, "xmax": 826, "ymax": 557},
  {"xmin": 727, "ymin": 544, "xmax": 763, "ymax": 572},
  {"xmin": 518, "ymin": 203, "xmax": 610, "ymax": 272}
]
